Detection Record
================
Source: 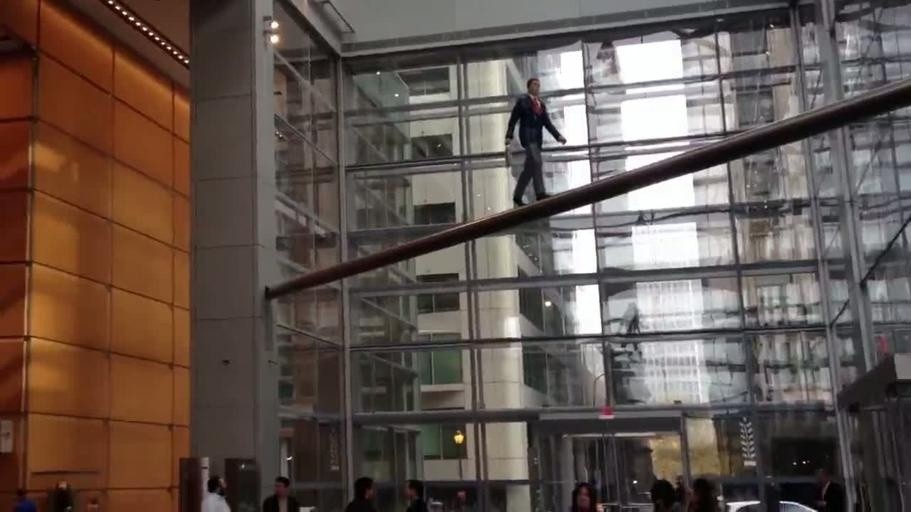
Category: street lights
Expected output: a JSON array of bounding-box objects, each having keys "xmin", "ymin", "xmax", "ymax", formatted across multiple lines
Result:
[{"xmin": 453, "ymin": 430, "xmax": 464, "ymax": 480}]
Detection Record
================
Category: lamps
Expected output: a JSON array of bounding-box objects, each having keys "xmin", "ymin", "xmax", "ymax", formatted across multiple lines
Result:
[{"xmin": 262, "ymin": 16, "xmax": 280, "ymax": 46}]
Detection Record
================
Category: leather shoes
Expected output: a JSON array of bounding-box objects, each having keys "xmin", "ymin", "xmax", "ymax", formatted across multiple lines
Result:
[
  {"xmin": 536, "ymin": 194, "xmax": 551, "ymax": 200},
  {"xmin": 513, "ymin": 196, "xmax": 526, "ymax": 206}
]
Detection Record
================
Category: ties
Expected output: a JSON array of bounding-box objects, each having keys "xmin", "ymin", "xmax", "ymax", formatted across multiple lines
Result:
[{"xmin": 532, "ymin": 96, "xmax": 539, "ymax": 111}]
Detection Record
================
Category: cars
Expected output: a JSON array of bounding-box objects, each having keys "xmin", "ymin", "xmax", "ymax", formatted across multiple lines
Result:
[{"xmin": 724, "ymin": 500, "xmax": 818, "ymax": 512}]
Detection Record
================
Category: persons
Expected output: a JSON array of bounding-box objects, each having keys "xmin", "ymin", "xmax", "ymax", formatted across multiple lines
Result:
[
  {"xmin": 569, "ymin": 480, "xmax": 604, "ymax": 511},
  {"xmin": 648, "ymin": 466, "xmax": 848, "ymax": 512},
  {"xmin": 403, "ymin": 478, "xmax": 431, "ymax": 511},
  {"xmin": 344, "ymin": 476, "xmax": 378, "ymax": 511},
  {"xmin": 47, "ymin": 480, "xmax": 74, "ymax": 510},
  {"xmin": 12, "ymin": 487, "xmax": 37, "ymax": 511},
  {"xmin": 505, "ymin": 76, "xmax": 571, "ymax": 209},
  {"xmin": 201, "ymin": 473, "xmax": 234, "ymax": 512},
  {"xmin": 261, "ymin": 476, "xmax": 301, "ymax": 511}
]
